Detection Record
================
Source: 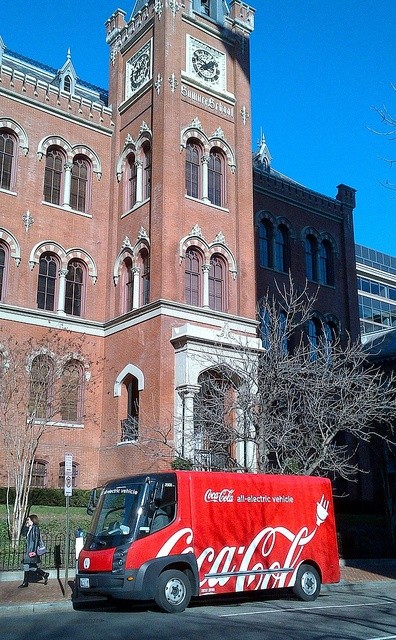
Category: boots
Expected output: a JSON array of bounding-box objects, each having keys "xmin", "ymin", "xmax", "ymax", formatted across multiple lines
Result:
[
  {"xmin": 36, "ymin": 568, "xmax": 49, "ymax": 585},
  {"xmin": 18, "ymin": 571, "xmax": 28, "ymax": 588}
]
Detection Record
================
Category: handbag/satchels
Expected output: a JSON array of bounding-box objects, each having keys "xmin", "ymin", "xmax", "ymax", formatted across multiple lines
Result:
[{"xmin": 37, "ymin": 545, "xmax": 47, "ymax": 556}]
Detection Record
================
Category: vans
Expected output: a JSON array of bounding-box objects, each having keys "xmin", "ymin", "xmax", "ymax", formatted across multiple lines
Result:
[{"xmin": 74, "ymin": 471, "xmax": 342, "ymax": 611}]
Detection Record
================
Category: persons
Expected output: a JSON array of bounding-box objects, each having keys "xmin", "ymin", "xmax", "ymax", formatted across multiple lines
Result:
[
  {"xmin": 146, "ymin": 498, "xmax": 169, "ymax": 534},
  {"xmin": 18, "ymin": 513, "xmax": 50, "ymax": 588}
]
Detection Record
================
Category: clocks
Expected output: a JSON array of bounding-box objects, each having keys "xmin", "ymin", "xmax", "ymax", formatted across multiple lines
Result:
[
  {"xmin": 191, "ymin": 47, "xmax": 219, "ymax": 79},
  {"xmin": 128, "ymin": 50, "xmax": 151, "ymax": 89}
]
